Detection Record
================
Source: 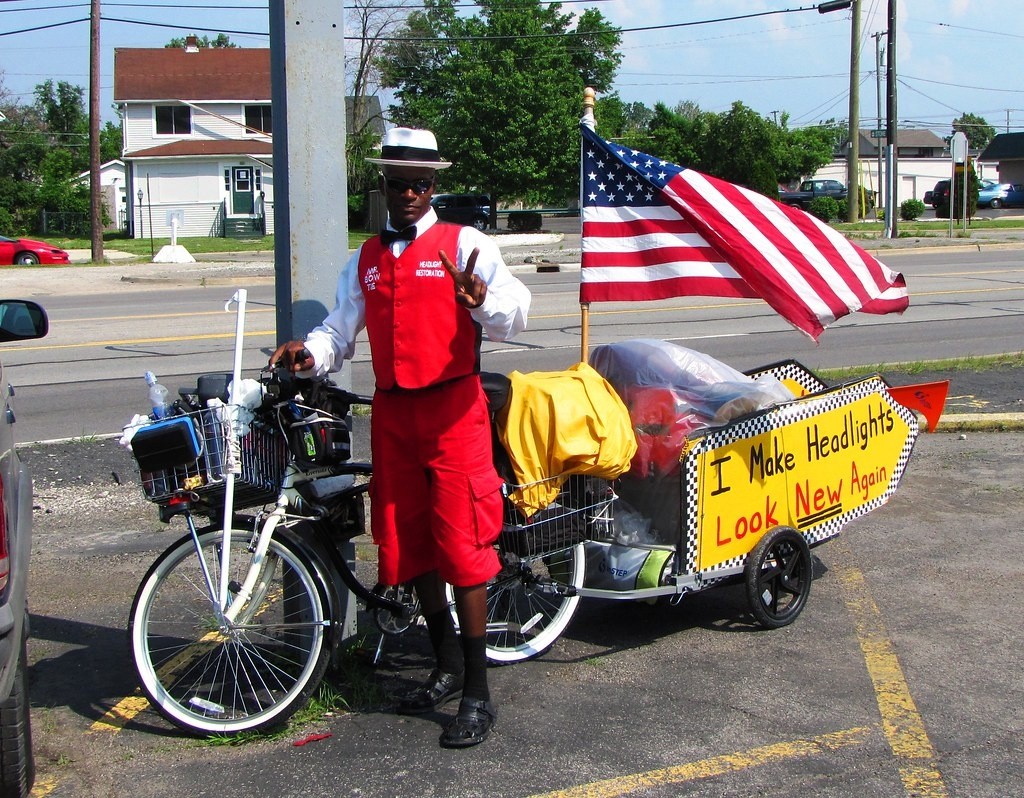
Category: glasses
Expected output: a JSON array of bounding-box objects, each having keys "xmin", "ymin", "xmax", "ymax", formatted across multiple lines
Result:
[{"xmin": 382, "ymin": 171, "xmax": 436, "ymax": 195}]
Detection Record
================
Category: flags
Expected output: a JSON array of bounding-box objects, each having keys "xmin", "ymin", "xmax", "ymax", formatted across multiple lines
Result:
[{"xmin": 578, "ymin": 113, "xmax": 909, "ymax": 344}]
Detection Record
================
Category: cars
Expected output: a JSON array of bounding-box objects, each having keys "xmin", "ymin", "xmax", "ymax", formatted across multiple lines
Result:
[
  {"xmin": 922, "ymin": 176, "xmax": 1024, "ymax": 211},
  {"xmin": 777, "ymin": 179, "xmax": 878, "ymax": 219},
  {"xmin": 0, "ymin": 296, "xmax": 53, "ymax": 798},
  {"xmin": 1, "ymin": 234, "xmax": 72, "ymax": 267}
]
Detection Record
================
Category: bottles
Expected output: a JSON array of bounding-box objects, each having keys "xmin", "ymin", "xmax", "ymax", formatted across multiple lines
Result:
[
  {"xmin": 287, "ymin": 398, "xmax": 312, "ymax": 432},
  {"xmin": 202, "ymin": 405, "xmax": 226, "ymax": 480},
  {"xmin": 141, "ymin": 370, "xmax": 176, "ymax": 420}
]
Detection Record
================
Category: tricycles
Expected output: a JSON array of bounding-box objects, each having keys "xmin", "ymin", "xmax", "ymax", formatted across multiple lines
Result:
[{"xmin": 129, "ymin": 342, "xmax": 917, "ymax": 743}]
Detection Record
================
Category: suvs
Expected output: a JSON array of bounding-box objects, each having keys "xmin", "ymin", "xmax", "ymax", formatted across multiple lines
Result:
[{"xmin": 431, "ymin": 194, "xmax": 493, "ymax": 230}]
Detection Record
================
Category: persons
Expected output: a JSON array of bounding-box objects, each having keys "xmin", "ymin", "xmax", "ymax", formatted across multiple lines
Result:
[{"xmin": 265, "ymin": 127, "xmax": 533, "ymax": 748}]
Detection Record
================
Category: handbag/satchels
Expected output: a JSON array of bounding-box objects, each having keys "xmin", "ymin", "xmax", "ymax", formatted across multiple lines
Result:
[{"xmin": 289, "ymin": 417, "xmax": 351, "ymax": 470}]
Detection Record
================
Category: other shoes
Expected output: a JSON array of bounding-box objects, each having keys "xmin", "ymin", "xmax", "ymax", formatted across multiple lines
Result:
[
  {"xmin": 439, "ymin": 697, "xmax": 497, "ymax": 746},
  {"xmin": 407, "ymin": 666, "xmax": 465, "ymax": 713}
]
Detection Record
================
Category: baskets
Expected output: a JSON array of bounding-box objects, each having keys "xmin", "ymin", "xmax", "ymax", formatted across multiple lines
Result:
[
  {"xmin": 491, "ymin": 470, "xmax": 620, "ymax": 561},
  {"xmin": 120, "ymin": 403, "xmax": 288, "ymax": 506}
]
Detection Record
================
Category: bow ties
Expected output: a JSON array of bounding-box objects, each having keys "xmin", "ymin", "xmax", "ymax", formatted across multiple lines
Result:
[{"xmin": 379, "ymin": 225, "xmax": 417, "ymax": 245}]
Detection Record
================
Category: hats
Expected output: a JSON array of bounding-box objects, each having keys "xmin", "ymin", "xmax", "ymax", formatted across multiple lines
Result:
[{"xmin": 364, "ymin": 128, "xmax": 452, "ymax": 170}]
